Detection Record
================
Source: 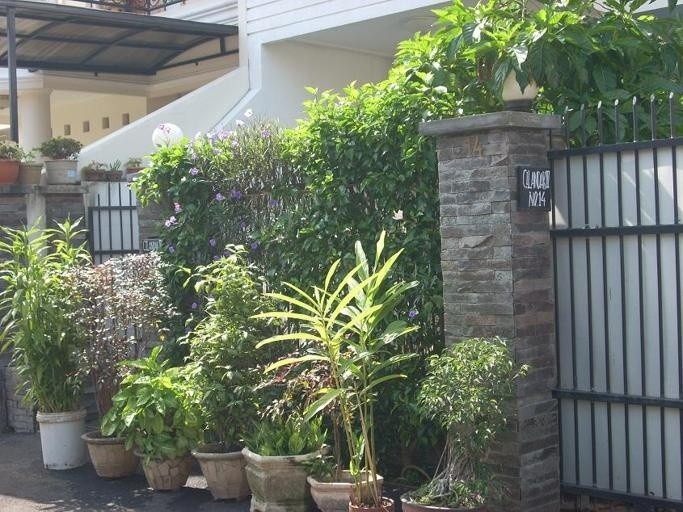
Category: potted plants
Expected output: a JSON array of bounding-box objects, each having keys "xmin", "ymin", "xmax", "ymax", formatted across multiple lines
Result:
[
  {"xmin": 310, "ymin": 295, "xmax": 395, "ymax": 510},
  {"xmin": 0, "ymin": 135, "xmax": 145, "ymax": 185},
  {"xmin": 400, "ymin": 338, "xmax": 533, "ymax": 510},
  {"xmin": 248, "ymin": 227, "xmax": 420, "ymax": 512},
  {"xmin": 103, "ymin": 346, "xmax": 202, "ymax": 490},
  {"xmin": 172, "ymin": 244, "xmax": 298, "ymax": 502},
  {"xmin": 60, "ymin": 244, "xmax": 172, "ymax": 480},
  {"xmin": 238, "ymin": 412, "xmax": 328, "ymax": 510},
  {"xmin": 0, "ymin": 212, "xmax": 96, "ymax": 469}
]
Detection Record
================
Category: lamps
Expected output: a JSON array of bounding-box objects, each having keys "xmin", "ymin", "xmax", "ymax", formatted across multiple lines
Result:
[
  {"xmin": 153, "ymin": 123, "xmax": 185, "ymax": 153},
  {"xmin": 503, "ymin": 57, "xmax": 538, "ymax": 114}
]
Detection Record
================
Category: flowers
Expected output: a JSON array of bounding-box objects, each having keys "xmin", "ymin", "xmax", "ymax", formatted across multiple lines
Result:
[{"xmin": 161, "ymin": 214, "xmax": 430, "ymax": 315}]
